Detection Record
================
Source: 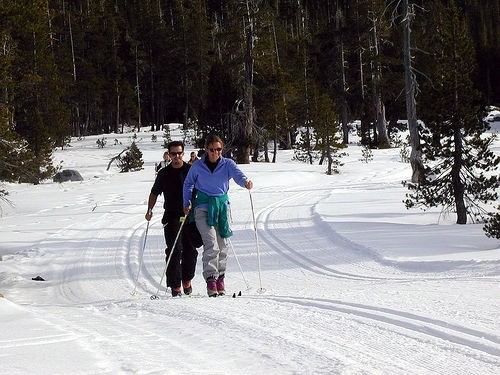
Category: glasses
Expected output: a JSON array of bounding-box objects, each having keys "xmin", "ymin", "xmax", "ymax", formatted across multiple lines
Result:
[
  {"xmin": 191, "ymin": 156, "xmax": 194, "ymax": 157},
  {"xmin": 209, "ymin": 148, "xmax": 222, "ymax": 151},
  {"xmin": 170, "ymin": 152, "xmax": 182, "ymax": 155}
]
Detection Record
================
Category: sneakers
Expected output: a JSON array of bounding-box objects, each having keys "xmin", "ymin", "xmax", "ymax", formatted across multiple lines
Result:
[
  {"xmin": 216, "ymin": 278, "xmax": 225, "ymax": 294},
  {"xmin": 172, "ymin": 287, "xmax": 182, "ymax": 296},
  {"xmin": 206, "ymin": 280, "xmax": 218, "ymax": 296},
  {"xmin": 183, "ymin": 280, "xmax": 192, "ymax": 294}
]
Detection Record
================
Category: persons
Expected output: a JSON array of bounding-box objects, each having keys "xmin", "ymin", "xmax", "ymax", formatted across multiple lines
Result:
[
  {"xmin": 145, "ymin": 141, "xmax": 198, "ymax": 297},
  {"xmin": 183, "ymin": 138, "xmax": 253, "ymax": 296},
  {"xmin": 155, "ymin": 152, "xmax": 172, "ymax": 175},
  {"xmin": 187, "ymin": 152, "xmax": 199, "ymax": 165}
]
